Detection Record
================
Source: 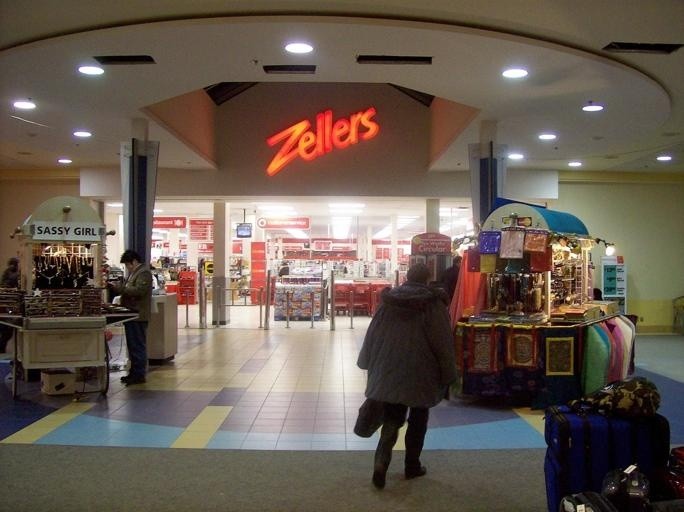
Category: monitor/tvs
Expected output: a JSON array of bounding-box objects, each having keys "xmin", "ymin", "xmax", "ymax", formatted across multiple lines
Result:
[{"xmin": 237, "ymin": 226, "xmax": 251, "ymax": 237}]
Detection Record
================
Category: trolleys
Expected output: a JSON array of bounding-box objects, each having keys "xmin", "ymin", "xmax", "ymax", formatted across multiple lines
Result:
[{"xmin": 333, "ymin": 277, "xmax": 391, "ymax": 317}]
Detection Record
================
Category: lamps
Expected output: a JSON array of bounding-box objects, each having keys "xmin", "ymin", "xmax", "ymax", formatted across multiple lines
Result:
[
  {"xmin": 552, "ymin": 233, "xmax": 579, "ymax": 251},
  {"xmin": 593, "ymin": 235, "xmax": 617, "ymax": 259}
]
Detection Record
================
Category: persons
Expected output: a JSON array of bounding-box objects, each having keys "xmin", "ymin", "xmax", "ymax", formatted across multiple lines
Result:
[
  {"xmin": 162, "ymin": 257, "xmax": 169, "ymax": 268},
  {"xmin": 436, "ymin": 256, "xmax": 462, "ymax": 302},
  {"xmin": 0, "ymin": 257, "xmax": 21, "ymax": 354},
  {"xmin": 593, "ymin": 288, "xmax": 603, "ymax": 301},
  {"xmin": 109, "ymin": 248, "xmax": 152, "ymax": 387},
  {"xmin": 356, "ymin": 262, "xmax": 456, "ymax": 491},
  {"xmin": 198, "ymin": 258, "xmax": 205, "ymax": 272},
  {"xmin": 278, "ymin": 262, "xmax": 290, "ymax": 276}
]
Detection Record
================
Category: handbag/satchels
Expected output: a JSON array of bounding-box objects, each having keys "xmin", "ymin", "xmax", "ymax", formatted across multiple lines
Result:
[{"xmin": 354, "ymin": 393, "xmax": 383, "ymax": 439}]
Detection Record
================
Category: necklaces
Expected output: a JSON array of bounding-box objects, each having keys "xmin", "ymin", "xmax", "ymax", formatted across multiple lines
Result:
[{"xmin": 31, "ymin": 243, "xmax": 93, "ymax": 288}]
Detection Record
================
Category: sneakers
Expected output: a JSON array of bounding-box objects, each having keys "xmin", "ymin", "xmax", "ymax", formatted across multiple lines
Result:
[
  {"xmin": 403, "ymin": 465, "xmax": 428, "ymax": 480},
  {"xmin": 369, "ymin": 466, "xmax": 385, "ymax": 491},
  {"xmin": 119, "ymin": 371, "xmax": 146, "ymax": 385}
]
